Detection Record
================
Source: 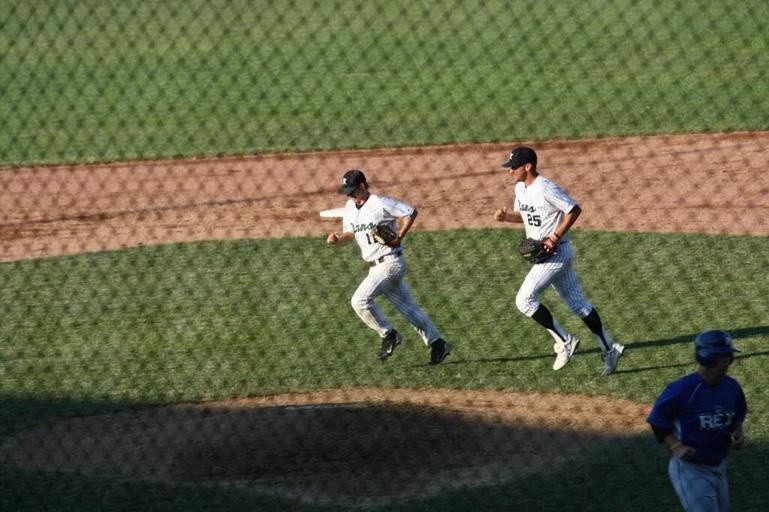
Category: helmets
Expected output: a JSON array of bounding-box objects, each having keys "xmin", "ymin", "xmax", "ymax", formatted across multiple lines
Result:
[{"xmin": 694, "ymin": 329, "xmax": 741, "ymax": 361}]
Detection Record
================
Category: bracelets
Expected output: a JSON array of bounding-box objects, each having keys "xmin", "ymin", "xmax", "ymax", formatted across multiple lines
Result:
[{"xmin": 669, "ymin": 441, "xmax": 682, "ymax": 451}]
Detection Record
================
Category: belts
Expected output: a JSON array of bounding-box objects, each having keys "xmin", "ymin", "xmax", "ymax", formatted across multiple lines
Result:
[
  {"xmin": 556, "ymin": 240, "xmax": 569, "ymax": 245},
  {"xmin": 685, "ymin": 455, "xmax": 724, "ymax": 469},
  {"xmin": 368, "ymin": 250, "xmax": 403, "ymax": 267}
]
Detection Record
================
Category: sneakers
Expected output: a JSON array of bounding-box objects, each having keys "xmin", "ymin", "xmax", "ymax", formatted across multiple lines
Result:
[
  {"xmin": 553, "ymin": 333, "xmax": 580, "ymax": 371},
  {"xmin": 600, "ymin": 342, "xmax": 625, "ymax": 376},
  {"xmin": 377, "ymin": 329, "xmax": 402, "ymax": 361},
  {"xmin": 428, "ymin": 337, "xmax": 452, "ymax": 366}
]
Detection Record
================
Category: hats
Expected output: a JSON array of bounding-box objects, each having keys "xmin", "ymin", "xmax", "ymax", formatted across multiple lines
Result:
[
  {"xmin": 501, "ymin": 146, "xmax": 537, "ymax": 170},
  {"xmin": 338, "ymin": 170, "xmax": 367, "ymax": 195}
]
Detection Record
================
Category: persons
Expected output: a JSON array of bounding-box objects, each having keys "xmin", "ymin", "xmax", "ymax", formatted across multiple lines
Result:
[
  {"xmin": 644, "ymin": 328, "xmax": 748, "ymax": 512},
  {"xmin": 494, "ymin": 146, "xmax": 625, "ymax": 374},
  {"xmin": 326, "ymin": 168, "xmax": 454, "ymax": 365}
]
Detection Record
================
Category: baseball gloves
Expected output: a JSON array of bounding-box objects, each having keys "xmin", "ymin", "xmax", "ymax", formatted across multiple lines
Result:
[
  {"xmin": 371, "ymin": 225, "xmax": 401, "ymax": 248},
  {"xmin": 519, "ymin": 237, "xmax": 557, "ymax": 264}
]
